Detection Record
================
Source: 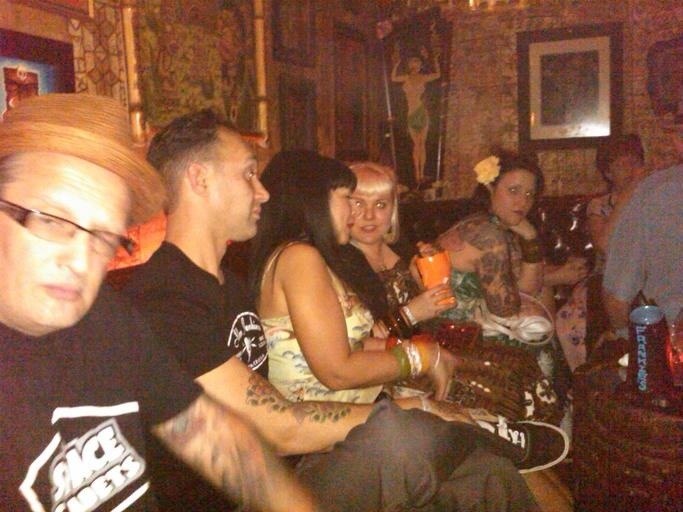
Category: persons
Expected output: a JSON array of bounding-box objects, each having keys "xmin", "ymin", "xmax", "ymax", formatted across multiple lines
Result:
[{"xmin": 391, "ymin": 53, "xmax": 441, "ymax": 187}]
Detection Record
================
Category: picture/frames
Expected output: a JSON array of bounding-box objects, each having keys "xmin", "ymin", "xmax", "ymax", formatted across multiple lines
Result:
[
  {"xmin": 515, "ymin": 20, "xmax": 621, "ymax": 149},
  {"xmin": 0, "ymin": 27, "xmax": 76, "ymax": 123},
  {"xmin": 273, "ymin": 0, "xmax": 373, "ymax": 164}
]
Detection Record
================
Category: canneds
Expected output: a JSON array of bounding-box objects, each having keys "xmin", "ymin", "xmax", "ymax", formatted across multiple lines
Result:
[{"xmin": 627, "ymin": 304, "xmax": 668, "ymax": 396}]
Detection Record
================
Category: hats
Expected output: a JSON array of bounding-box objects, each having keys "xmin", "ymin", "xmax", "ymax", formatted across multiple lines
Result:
[{"xmin": 0, "ymin": 91, "xmax": 172, "ymax": 233}]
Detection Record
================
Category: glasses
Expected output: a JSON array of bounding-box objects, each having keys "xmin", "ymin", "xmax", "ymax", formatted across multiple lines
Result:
[{"xmin": 0, "ymin": 197, "xmax": 140, "ymax": 262}]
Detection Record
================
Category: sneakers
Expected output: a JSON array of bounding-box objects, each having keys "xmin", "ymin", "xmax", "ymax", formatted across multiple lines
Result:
[{"xmin": 468, "ymin": 413, "xmax": 571, "ymax": 479}]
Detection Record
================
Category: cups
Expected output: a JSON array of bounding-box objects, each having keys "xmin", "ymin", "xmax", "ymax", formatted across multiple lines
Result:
[{"xmin": 416, "ymin": 246, "xmax": 457, "ymax": 311}]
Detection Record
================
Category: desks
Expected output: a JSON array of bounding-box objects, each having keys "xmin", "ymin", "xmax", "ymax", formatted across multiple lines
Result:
[{"xmin": 574, "ymin": 360, "xmax": 683, "ymax": 512}]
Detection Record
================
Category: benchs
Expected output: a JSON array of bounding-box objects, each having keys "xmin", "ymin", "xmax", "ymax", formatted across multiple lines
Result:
[{"xmin": 218, "ymin": 192, "xmax": 604, "ymax": 316}]
